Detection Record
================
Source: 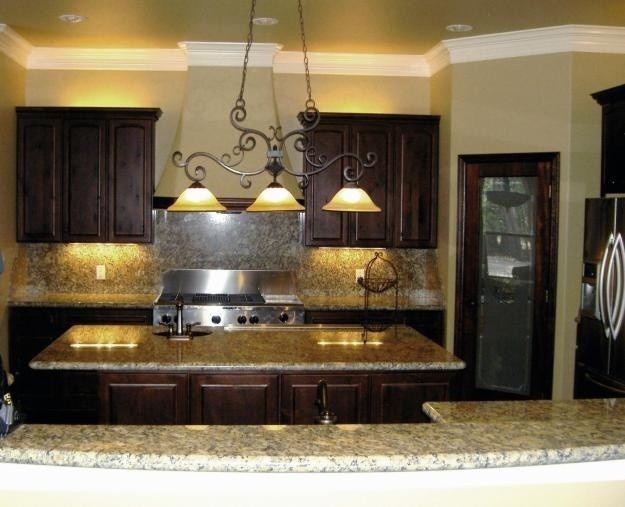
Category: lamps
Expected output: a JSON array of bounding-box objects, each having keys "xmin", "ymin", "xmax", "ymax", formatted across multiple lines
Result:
[{"xmin": 157, "ymin": 1, "xmax": 385, "ymax": 215}]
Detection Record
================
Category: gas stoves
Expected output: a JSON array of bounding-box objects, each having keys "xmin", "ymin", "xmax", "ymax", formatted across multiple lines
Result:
[{"xmin": 158, "ymin": 292, "xmax": 304, "ymax": 306}]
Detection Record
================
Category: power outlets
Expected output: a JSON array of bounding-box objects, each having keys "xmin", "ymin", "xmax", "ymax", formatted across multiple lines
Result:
[{"xmin": 356, "ymin": 269, "xmax": 365, "ymax": 283}]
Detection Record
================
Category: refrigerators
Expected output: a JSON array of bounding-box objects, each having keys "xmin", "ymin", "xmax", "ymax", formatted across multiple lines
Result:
[{"xmin": 573, "ymin": 196, "xmax": 624, "ymax": 400}]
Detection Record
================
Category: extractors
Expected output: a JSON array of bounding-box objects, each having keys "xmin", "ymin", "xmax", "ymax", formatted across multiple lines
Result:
[{"xmin": 153, "ymin": 197, "xmax": 305, "ymax": 214}]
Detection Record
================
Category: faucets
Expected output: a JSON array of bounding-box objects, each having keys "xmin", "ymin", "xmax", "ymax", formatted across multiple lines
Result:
[{"xmin": 175, "ymin": 295, "xmax": 183, "ymax": 335}]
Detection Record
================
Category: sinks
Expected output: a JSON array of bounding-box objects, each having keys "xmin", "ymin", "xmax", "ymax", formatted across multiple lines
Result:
[{"xmin": 155, "ymin": 331, "xmax": 211, "ymax": 336}]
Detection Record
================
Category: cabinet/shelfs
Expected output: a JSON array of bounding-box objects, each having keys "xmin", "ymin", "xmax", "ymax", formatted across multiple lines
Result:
[
  {"xmin": 16, "ymin": 106, "xmax": 61, "ymax": 244},
  {"xmin": 64, "ymin": 107, "xmax": 163, "ymax": 246},
  {"xmin": 60, "ymin": 371, "xmax": 456, "ymax": 423},
  {"xmin": 297, "ymin": 113, "xmax": 395, "ymax": 247},
  {"xmin": 397, "ymin": 113, "xmax": 441, "ymax": 249},
  {"xmin": 305, "ymin": 311, "xmax": 446, "ymax": 348},
  {"xmin": 9, "ymin": 310, "xmax": 150, "ymax": 382}
]
